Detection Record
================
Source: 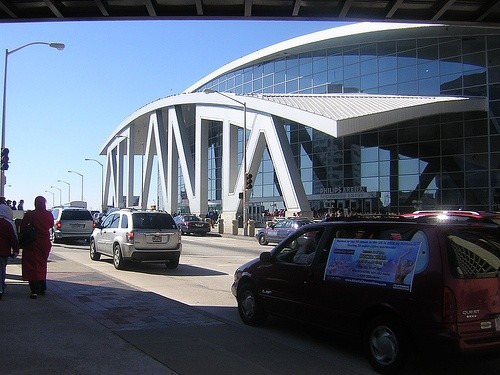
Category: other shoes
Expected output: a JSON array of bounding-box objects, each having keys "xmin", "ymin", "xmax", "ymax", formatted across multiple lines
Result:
[
  {"xmin": 0, "ymin": 289, "xmax": 4, "ymax": 295},
  {"xmin": 31, "ymin": 292, "xmax": 38, "ymax": 298}
]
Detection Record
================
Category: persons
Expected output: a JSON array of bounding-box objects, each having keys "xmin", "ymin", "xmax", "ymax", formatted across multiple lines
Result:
[
  {"xmin": 7, "ymin": 200, "xmax": 24, "ymax": 211},
  {"xmin": 293, "ymin": 212, "xmax": 300, "ymax": 217},
  {"xmin": 264, "ymin": 208, "xmax": 285, "ymax": 217},
  {"xmin": 198, "ymin": 209, "xmax": 218, "ymax": 228},
  {"xmin": 0, "ymin": 197, "xmax": 19, "ymax": 294},
  {"xmin": 294, "ymin": 237, "xmax": 317, "ymax": 265},
  {"xmin": 171, "ymin": 211, "xmax": 180, "ymax": 218},
  {"xmin": 313, "ymin": 209, "xmax": 344, "ymax": 219},
  {"xmin": 395, "ymin": 250, "xmax": 415, "ymax": 283},
  {"xmin": 379, "ymin": 205, "xmax": 386, "ymax": 214},
  {"xmin": 20, "ymin": 196, "xmax": 54, "ymax": 297}
]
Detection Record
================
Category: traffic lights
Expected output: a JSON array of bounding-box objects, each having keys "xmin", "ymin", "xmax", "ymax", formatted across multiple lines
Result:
[
  {"xmin": 246, "ymin": 173, "xmax": 253, "ymax": 189},
  {"xmin": 0, "ymin": 147, "xmax": 9, "ymax": 170}
]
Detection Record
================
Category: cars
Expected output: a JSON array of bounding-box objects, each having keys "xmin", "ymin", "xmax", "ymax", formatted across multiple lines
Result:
[
  {"xmin": 256, "ymin": 217, "xmax": 316, "ymax": 250},
  {"xmin": 232, "ymin": 211, "xmax": 500, "ymax": 374},
  {"xmin": 173, "ymin": 214, "xmax": 211, "ymax": 236},
  {"xmin": 50, "ymin": 207, "xmax": 96, "ymax": 243}
]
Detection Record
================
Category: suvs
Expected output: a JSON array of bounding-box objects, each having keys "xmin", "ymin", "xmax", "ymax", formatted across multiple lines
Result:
[
  {"xmin": 89, "ymin": 206, "xmax": 184, "ymax": 270},
  {"xmin": 400, "ymin": 208, "xmax": 500, "ymax": 224}
]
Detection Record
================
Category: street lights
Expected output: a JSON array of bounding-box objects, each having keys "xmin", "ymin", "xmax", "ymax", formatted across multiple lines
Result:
[
  {"xmin": 51, "ymin": 186, "xmax": 62, "ymax": 206},
  {"xmin": 67, "ymin": 170, "xmax": 84, "ymax": 201},
  {"xmin": 58, "ymin": 180, "xmax": 71, "ymax": 200},
  {"xmin": 44, "ymin": 190, "xmax": 54, "ymax": 206},
  {"xmin": 85, "ymin": 158, "xmax": 104, "ymax": 214},
  {"xmin": 0, "ymin": 41, "xmax": 67, "ymax": 201},
  {"xmin": 203, "ymin": 87, "xmax": 250, "ymax": 236}
]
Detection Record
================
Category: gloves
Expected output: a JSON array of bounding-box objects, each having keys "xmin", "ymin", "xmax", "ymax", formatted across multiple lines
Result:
[{"xmin": 10, "ymin": 252, "xmax": 18, "ymax": 259}]
